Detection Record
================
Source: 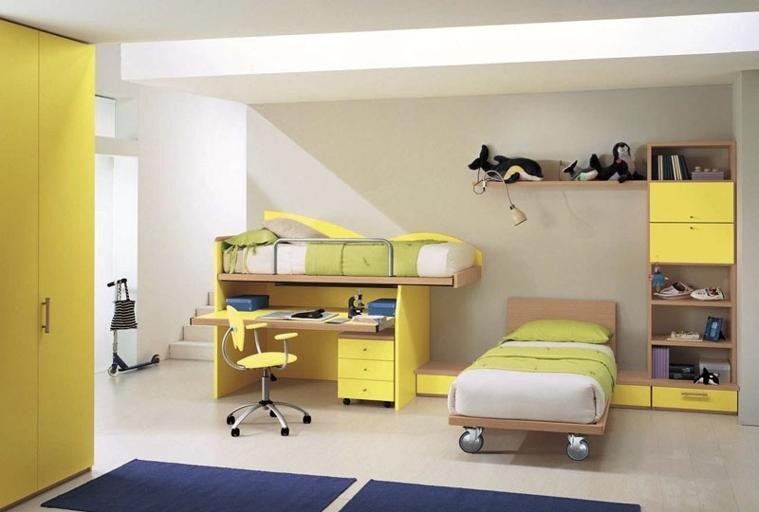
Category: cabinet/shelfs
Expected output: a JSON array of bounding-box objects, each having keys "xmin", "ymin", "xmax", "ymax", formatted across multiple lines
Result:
[
  {"xmin": 645, "ymin": 139, "xmax": 740, "ymax": 414},
  {"xmin": 0, "ymin": 17, "xmax": 95, "ymax": 511},
  {"xmin": 334, "ymin": 332, "xmax": 395, "ymax": 410}
]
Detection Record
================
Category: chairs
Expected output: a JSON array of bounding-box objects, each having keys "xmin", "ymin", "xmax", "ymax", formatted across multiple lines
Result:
[{"xmin": 220, "ymin": 305, "xmax": 312, "ymax": 439}]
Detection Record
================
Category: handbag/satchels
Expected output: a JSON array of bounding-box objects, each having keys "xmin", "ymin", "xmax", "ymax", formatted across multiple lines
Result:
[{"xmin": 111, "ymin": 279, "xmax": 138, "ymax": 330}]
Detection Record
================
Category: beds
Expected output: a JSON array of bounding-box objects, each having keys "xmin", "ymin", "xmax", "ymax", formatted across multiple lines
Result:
[
  {"xmin": 216, "ymin": 235, "xmax": 482, "ymax": 287},
  {"xmin": 446, "ymin": 295, "xmax": 621, "ymax": 460}
]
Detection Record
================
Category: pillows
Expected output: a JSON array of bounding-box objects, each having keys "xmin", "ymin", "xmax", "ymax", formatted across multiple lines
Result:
[{"xmin": 498, "ymin": 317, "xmax": 613, "ymax": 344}]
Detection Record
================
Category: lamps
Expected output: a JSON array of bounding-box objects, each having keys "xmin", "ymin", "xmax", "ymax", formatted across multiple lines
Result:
[{"xmin": 472, "ymin": 169, "xmax": 530, "ymax": 227}]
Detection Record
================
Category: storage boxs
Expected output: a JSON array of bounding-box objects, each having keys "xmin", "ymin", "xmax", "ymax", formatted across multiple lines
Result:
[
  {"xmin": 367, "ymin": 298, "xmax": 396, "ymax": 315},
  {"xmin": 225, "ymin": 294, "xmax": 269, "ymax": 310},
  {"xmin": 699, "ymin": 357, "xmax": 731, "ymax": 385}
]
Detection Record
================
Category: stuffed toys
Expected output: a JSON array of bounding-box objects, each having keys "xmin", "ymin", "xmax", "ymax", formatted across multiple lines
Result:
[
  {"xmin": 468, "ymin": 144, "xmax": 545, "ymax": 184},
  {"xmin": 693, "ymin": 367, "xmax": 720, "ymax": 387},
  {"xmin": 561, "ymin": 142, "xmax": 645, "ymax": 184}
]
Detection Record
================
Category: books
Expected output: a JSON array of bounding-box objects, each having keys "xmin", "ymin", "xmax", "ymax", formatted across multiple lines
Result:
[
  {"xmin": 652, "ymin": 330, "xmax": 703, "ymax": 380},
  {"xmin": 652, "ymin": 153, "xmax": 691, "ymax": 180}
]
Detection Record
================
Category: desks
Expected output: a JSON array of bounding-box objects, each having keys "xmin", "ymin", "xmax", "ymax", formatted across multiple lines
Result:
[{"xmin": 188, "ymin": 304, "xmax": 395, "ymax": 400}]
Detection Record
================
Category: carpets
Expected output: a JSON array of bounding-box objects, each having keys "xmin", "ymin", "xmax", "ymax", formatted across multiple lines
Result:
[
  {"xmin": 338, "ymin": 479, "xmax": 641, "ymax": 512},
  {"xmin": 39, "ymin": 453, "xmax": 355, "ymax": 512}
]
